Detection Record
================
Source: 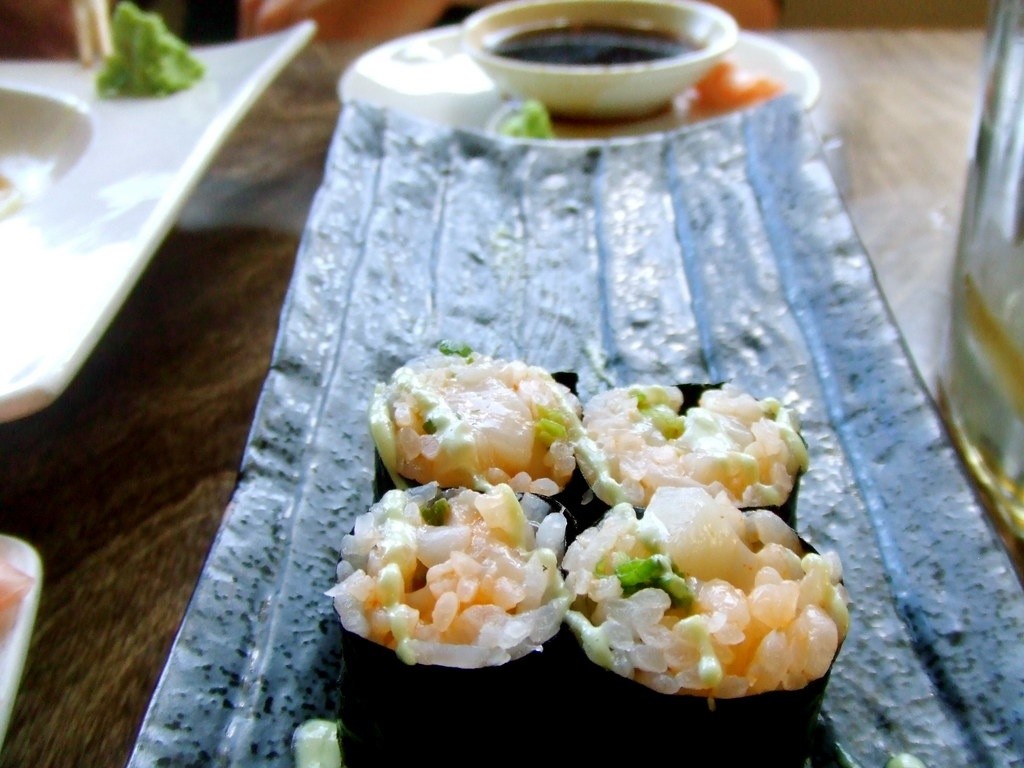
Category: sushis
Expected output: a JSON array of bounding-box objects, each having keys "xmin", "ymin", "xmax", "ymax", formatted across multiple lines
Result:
[{"xmin": 327, "ymin": 342, "xmax": 850, "ymax": 768}]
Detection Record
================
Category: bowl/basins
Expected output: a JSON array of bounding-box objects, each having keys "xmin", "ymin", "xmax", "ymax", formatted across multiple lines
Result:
[{"xmin": 462, "ymin": 0, "xmax": 739, "ymax": 119}]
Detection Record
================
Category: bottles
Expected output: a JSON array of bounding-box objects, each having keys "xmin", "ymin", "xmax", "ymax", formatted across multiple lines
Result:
[{"xmin": 940, "ymin": 4, "xmax": 1024, "ymax": 533}]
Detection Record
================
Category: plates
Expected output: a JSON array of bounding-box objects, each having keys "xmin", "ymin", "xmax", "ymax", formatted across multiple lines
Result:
[
  {"xmin": 0, "ymin": 534, "xmax": 46, "ymax": 748},
  {"xmin": 122, "ymin": 101, "xmax": 1024, "ymax": 768},
  {"xmin": 0, "ymin": 20, "xmax": 318, "ymax": 420},
  {"xmin": 338, "ymin": 20, "xmax": 817, "ymax": 140}
]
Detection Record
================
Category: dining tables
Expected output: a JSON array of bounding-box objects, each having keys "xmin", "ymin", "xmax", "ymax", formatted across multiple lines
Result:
[{"xmin": 0, "ymin": 31, "xmax": 1024, "ymax": 768}]
